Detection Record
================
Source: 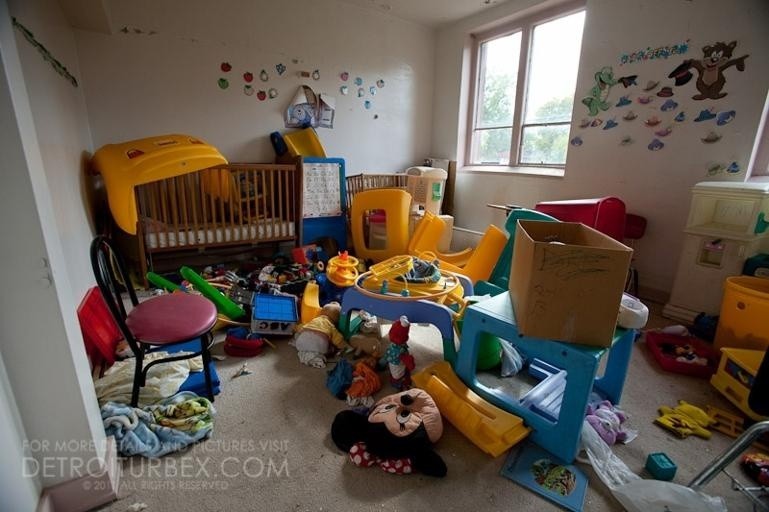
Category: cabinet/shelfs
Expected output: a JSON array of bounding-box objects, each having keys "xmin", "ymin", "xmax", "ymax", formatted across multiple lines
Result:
[{"xmin": 661, "ymin": 178, "xmax": 768, "ymax": 329}]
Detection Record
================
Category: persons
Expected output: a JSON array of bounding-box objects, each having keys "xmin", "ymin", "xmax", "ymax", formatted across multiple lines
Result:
[{"xmin": 290, "ymin": 297, "xmax": 450, "ymax": 482}]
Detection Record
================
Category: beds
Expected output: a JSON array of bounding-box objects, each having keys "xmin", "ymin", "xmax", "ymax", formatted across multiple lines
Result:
[
  {"xmin": 76, "ymin": 154, "xmax": 304, "ymax": 291},
  {"xmin": 343, "ymin": 171, "xmax": 409, "ymax": 221}
]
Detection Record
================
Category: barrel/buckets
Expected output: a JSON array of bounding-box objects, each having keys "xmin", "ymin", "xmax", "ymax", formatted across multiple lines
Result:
[{"xmin": 710, "ymin": 273, "xmax": 769, "ymax": 363}]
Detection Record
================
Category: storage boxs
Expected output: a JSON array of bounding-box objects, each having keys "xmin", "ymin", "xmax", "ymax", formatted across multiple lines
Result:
[{"xmin": 507, "ymin": 218, "xmax": 635, "ymax": 349}]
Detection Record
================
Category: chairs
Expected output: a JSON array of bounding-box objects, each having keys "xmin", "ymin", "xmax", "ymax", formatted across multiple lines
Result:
[
  {"xmin": 682, "ymin": 345, "xmax": 769, "ymax": 512},
  {"xmin": 88, "ymin": 236, "xmax": 219, "ymax": 406}
]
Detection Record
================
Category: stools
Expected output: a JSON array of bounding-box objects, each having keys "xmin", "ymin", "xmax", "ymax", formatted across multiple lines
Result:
[
  {"xmin": 456, "ymin": 284, "xmax": 637, "ymax": 463},
  {"xmin": 336, "ymin": 265, "xmax": 475, "ymax": 372}
]
